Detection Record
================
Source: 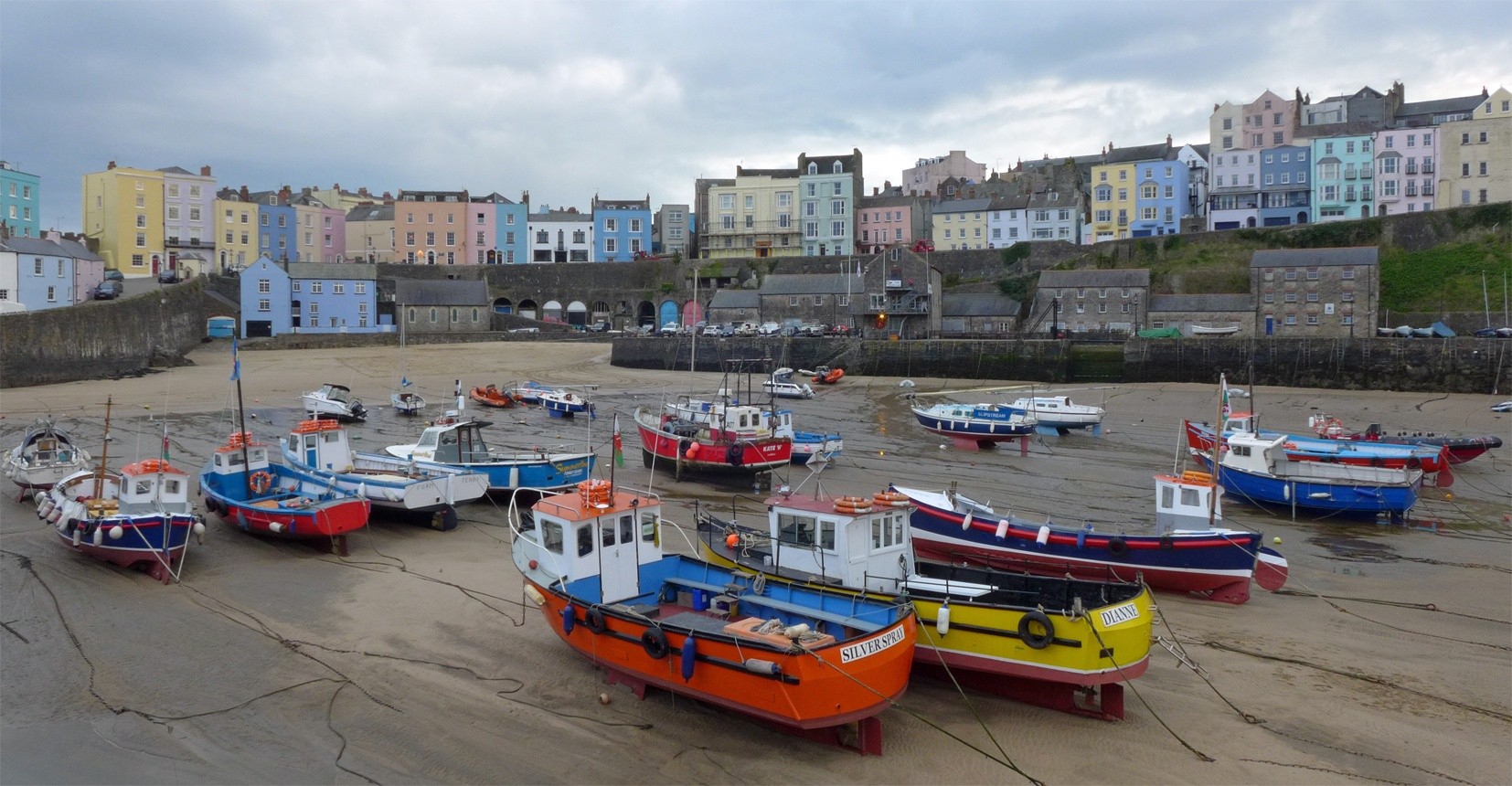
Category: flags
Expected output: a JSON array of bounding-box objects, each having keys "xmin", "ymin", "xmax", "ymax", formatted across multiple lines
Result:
[
  {"xmin": 229, "ymin": 335, "xmax": 242, "ymax": 381},
  {"xmin": 614, "ymin": 416, "xmax": 624, "ymax": 468},
  {"xmin": 1222, "ymin": 379, "xmax": 1232, "ymax": 422}
]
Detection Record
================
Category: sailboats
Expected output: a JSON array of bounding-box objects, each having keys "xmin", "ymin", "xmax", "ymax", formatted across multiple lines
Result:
[
  {"xmin": 633, "ymin": 268, "xmax": 846, "ymax": 484},
  {"xmin": 1473, "ymin": 269, "xmax": 1512, "ymax": 339},
  {"xmin": 393, "ymin": 301, "xmax": 427, "ymax": 417}
]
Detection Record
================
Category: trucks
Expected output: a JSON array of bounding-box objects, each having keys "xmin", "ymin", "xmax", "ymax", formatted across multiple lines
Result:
[
  {"xmin": 632, "ymin": 250, "xmax": 660, "ymax": 261},
  {"xmin": 910, "ymin": 239, "xmax": 936, "ymax": 253}
]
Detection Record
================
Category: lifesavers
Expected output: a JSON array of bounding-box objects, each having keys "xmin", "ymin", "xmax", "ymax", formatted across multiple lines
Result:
[
  {"xmin": 585, "ymin": 609, "xmax": 607, "ymax": 635},
  {"xmin": 578, "ymin": 479, "xmax": 615, "ymax": 507},
  {"xmin": 83, "ymin": 522, "xmax": 97, "ymax": 536},
  {"xmin": 295, "ymin": 419, "xmax": 342, "ymax": 432},
  {"xmin": 1181, "ymin": 469, "xmax": 1212, "ymax": 481},
  {"xmin": 215, "ymin": 501, "xmax": 228, "ymax": 517},
  {"xmin": 205, "ymin": 497, "xmax": 215, "ymax": 512},
  {"xmin": 1314, "ymin": 421, "xmax": 1326, "ymax": 434},
  {"xmin": 726, "ymin": 444, "xmax": 743, "ymax": 467},
  {"xmin": 1018, "ymin": 612, "xmax": 1055, "ymax": 650},
  {"xmin": 1320, "ymin": 456, "xmax": 1421, "ymax": 469},
  {"xmin": 219, "ymin": 431, "xmax": 262, "ymax": 450},
  {"xmin": 641, "ymin": 628, "xmax": 669, "ymax": 659},
  {"xmin": 68, "ymin": 519, "xmax": 78, "ymax": 535},
  {"xmin": 1160, "ymin": 537, "xmax": 1173, "ymax": 551},
  {"xmin": 250, "ymin": 471, "xmax": 271, "ymax": 493},
  {"xmin": 1327, "ymin": 418, "xmax": 1343, "ymax": 427},
  {"xmin": 873, "ymin": 492, "xmax": 911, "ymax": 506},
  {"xmin": 833, "ymin": 496, "xmax": 873, "ymax": 515},
  {"xmin": 679, "ymin": 440, "xmax": 690, "ymax": 456},
  {"xmin": 1107, "ymin": 537, "xmax": 1129, "ymax": 559},
  {"xmin": 141, "ymin": 459, "xmax": 169, "ymax": 471}
]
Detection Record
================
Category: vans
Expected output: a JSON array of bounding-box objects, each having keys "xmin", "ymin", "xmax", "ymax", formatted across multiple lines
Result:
[{"xmin": 228, "ymin": 263, "xmax": 248, "ymax": 278}]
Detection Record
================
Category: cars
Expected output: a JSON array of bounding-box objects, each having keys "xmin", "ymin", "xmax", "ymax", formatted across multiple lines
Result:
[
  {"xmin": 507, "ymin": 324, "xmax": 540, "ymax": 336},
  {"xmin": 158, "ymin": 268, "xmax": 184, "ymax": 284},
  {"xmin": 591, "ymin": 322, "xmax": 611, "ymax": 333},
  {"xmin": 94, "ymin": 281, "xmax": 124, "ymax": 300},
  {"xmin": 104, "ymin": 269, "xmax": 125, "ymax": 282}
]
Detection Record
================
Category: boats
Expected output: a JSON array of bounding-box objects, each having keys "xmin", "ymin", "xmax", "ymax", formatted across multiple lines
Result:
[
  {"xmin": 501, "ymin": 380, "xmax": 602, "ymax": 421},
  {"xmin": 691, "ymin": 430, "xmax": 1162, "ymax": 725},
  {"xmin": 887, "ymin": 374, "xmax": 1289, "ymax": 605},
  {"xmin": 300, "ymin": 383, "xmax": 370, "ymax": 427},
  {"xmin": 1184, "ymin": 356, "xmax": 1512, "ymax": 528},
  {"xmin": 1190, "ymin": 324, "xmax": 1240, "ymax": 336},
  {"xmin": 276, "ymin": 400, "xmax": 490, "ymax": 532},
  {"xmin": 469, "ymin": 384, "xmax": 511, "ymax": 408},
  {"xmin": 196, "ymin": 337, "xmax": 373, "ymax": 558},
  {"xmin": 382, "ymin": 377, "xmax": 599, "ymax": 508},
  {"xmin": 1376, "ymin": 320, "xmax": 1457, "ymax": 339},
  {"xmin": 34, "ymin": 392, "xmax": 209, "ymax": 588},
  {"xmin": 1, "ymin": 407, "xmax": 93, "ymax": 504},
  {"xmin": 507, "ymin": 411, "xmax": 920, "ymax": 758}
]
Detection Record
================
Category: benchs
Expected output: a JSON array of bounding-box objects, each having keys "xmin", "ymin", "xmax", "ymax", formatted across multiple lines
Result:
[{"xmin": 663, "ymin": 577, "xmax": 885, "ymax": 632}]
